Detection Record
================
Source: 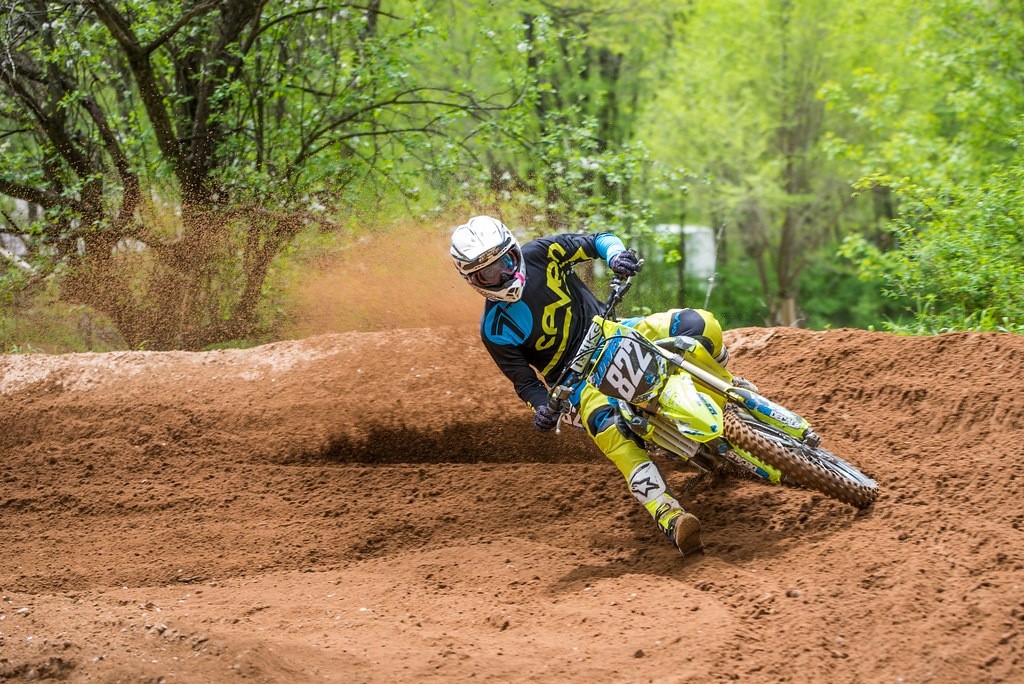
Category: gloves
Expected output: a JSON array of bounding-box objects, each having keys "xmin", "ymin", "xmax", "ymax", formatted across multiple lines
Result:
[
  {"xmin": 610, "ymin": 251, "xmax": 640, "ymax": 278},
  {"xmin": 534, "ymin": 404, "xmax": 563, "ymax": 432}
]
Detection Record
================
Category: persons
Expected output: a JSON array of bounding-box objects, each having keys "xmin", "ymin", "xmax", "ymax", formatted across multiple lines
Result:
[{"xmin": 449, "ymin": 213, "xmax": 760, "ymax": 556}]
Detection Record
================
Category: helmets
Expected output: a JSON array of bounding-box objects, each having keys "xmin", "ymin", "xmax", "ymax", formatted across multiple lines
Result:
[{"xmin": 450, "ymin": 217, "xmax": 526, "ymax": 302}]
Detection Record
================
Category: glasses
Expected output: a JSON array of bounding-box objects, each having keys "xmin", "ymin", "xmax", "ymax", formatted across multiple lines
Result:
[{"xmin": 460, "ymin": 244, "xmax": 520, "ymax": 284}]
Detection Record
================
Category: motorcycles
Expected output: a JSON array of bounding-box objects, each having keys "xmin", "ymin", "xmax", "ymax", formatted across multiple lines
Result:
[{"xmin": 544, "ymin": 250, "xmax": 880, "ymax": 509}]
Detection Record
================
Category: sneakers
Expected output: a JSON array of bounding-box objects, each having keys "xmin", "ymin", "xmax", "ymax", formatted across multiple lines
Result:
[
  {"xmin": 675, "ymin": 513, "xmax": 704, "ymax": 556},
  {"xmin": 729, "ymin": 377, "xmax": 758, "ymax": 393}
]
